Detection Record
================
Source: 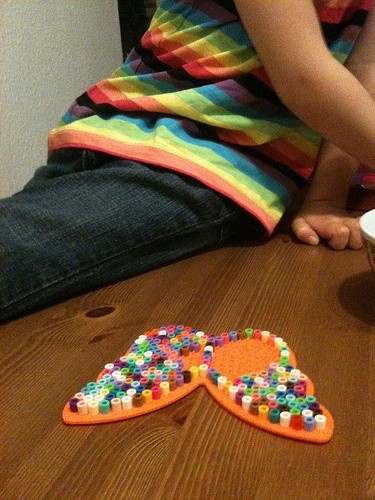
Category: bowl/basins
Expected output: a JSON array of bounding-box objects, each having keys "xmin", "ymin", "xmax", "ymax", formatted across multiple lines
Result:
[{"xmin": 359, "ymin": 208, "xmax": 375, "ymax": 275}]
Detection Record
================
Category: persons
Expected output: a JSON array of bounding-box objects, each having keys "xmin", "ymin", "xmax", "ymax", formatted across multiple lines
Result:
[{"xmin": 0, "ymin": 0, "xmax": 375, "ymax": 327}]
